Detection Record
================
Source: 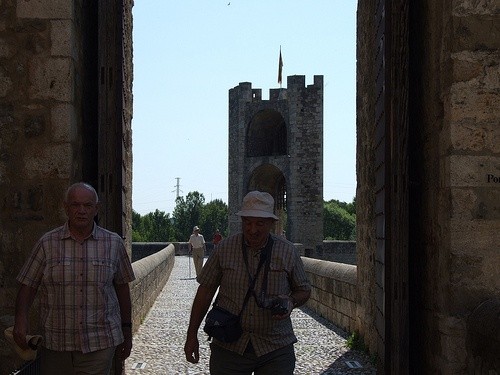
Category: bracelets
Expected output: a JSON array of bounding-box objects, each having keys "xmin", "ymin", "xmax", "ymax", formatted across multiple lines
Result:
[{"xmin": 292, "ymin": 295, "xmax": 298, "ymax": 307}]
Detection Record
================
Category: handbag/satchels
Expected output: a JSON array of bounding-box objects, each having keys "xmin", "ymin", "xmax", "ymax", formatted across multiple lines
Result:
[{"xmin": 203, "ymin": 235, "xmax": 272, "ymax": 343}]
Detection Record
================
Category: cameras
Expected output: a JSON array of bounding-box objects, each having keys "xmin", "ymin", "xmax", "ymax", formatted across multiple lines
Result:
[{"xmin": 270, "ymin": 299, "xmax": 289, "ymax": 316}]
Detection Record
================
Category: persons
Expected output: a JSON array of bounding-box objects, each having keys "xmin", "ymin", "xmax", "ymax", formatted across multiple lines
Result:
[
  {"xmin": 212, "ymin": 228, "xmax": 221, "ymax": 249},
  {"xmin": 184, "ymin": 191, "xmax": 311, "ymax": 375},
  {"xmin": 189, "ymin": 226, "xmax": 206, "ymax": 279},
  {"xmin": 16, "ymin": 182, "xmax": 134, "ymax": 375}
]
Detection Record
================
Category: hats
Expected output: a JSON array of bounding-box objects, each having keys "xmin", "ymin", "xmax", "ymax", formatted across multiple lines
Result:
[
  {"xmin": 235, "ymin": 190, "xmax": 280, "ymax": 222},
  {"xmin": 193, "ymin": 226, "xmax": 200, "ymax": 233},
  {"xmin": 4, "ymin": 325, "xmax": 45, "ymax": 362}
]
